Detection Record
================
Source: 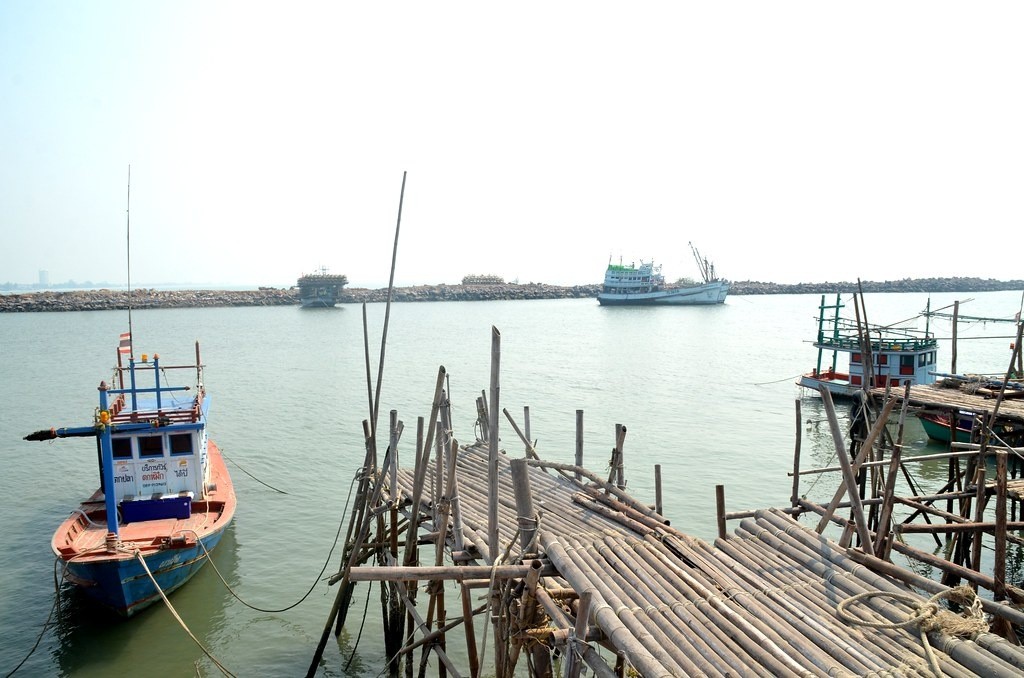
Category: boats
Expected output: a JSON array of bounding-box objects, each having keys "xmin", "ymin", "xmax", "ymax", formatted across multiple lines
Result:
[
  {"xmin": 796, "ymin": 313, "xmax": 937, "ymax": 405},
  {"xmin": 915, "ymin": 407, "xmax": 1024, "ymax": 451},
  {"xmin": 296, "ymin": 276, "xmax": 348, "ymax": 307},
  {"xmin": 23, "ymin": 341, "xmax": 237, "ymax": 620},
  {"xmin": 596, "ymin": 259, "xmax": 731, "ymax": 307}
]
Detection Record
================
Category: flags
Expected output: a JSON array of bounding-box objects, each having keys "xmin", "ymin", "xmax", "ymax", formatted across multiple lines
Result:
[{"xmin": 118, "ymin": 332, "xmax": 131, "ymax": 354}]
